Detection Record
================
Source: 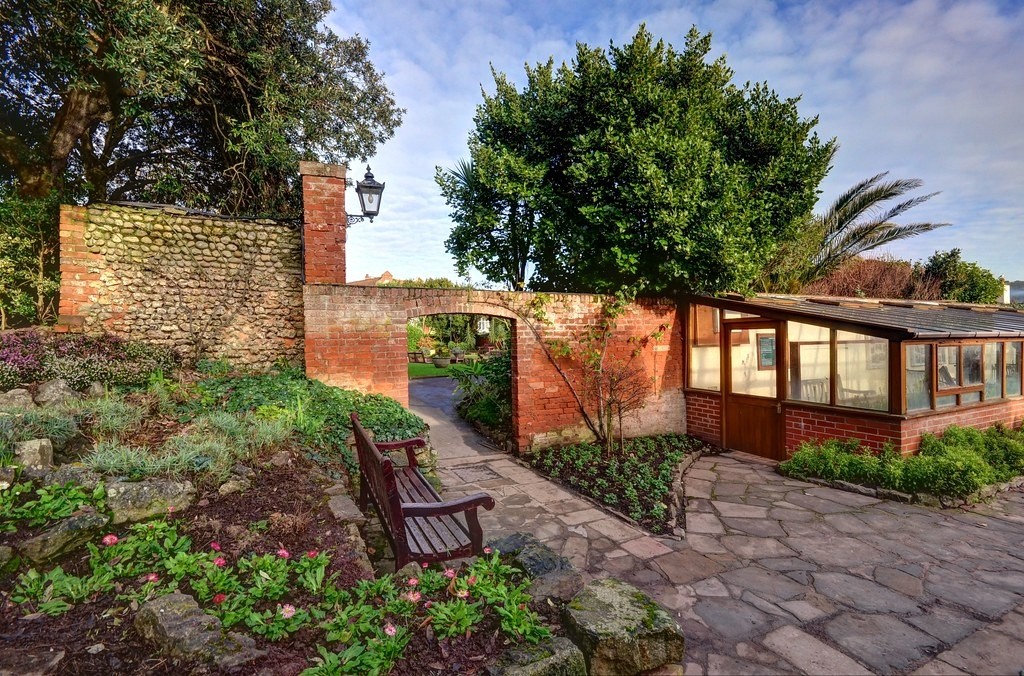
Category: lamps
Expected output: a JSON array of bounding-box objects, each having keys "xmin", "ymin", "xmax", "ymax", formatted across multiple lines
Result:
[{"xmin": 344, "ymin": 163, "xmax": 386, "ymax": 228}]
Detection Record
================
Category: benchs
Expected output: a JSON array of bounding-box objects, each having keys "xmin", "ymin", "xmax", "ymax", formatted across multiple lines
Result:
[
  {"xmin": 788, "ymin": 374, "xmax": 876, "ymax": 405},
  {"xmin": 349, "ymin": 410, "xmax": 496, "ymax": 576}
]
